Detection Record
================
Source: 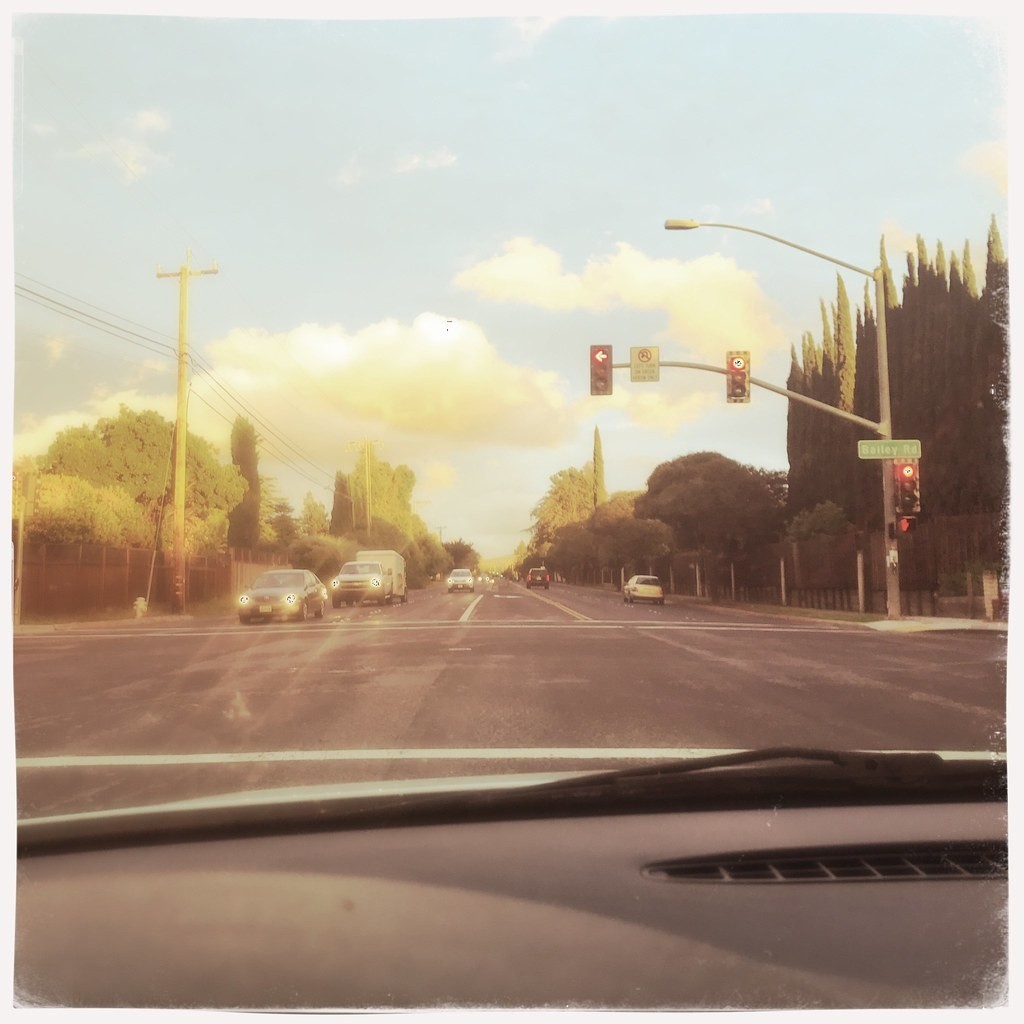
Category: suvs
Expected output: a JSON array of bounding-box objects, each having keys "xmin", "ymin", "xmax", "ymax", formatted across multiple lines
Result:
[
  {"xmin": 526, "ymin": 568, "xmax": 550, "ymax": 590},
  {"xmin": 329, "ymin": 560, "xmax": 391, "ymax": 609},
  {"xmin": 447, "ymin": 568, "xmax": 474, "ymax": 593}
]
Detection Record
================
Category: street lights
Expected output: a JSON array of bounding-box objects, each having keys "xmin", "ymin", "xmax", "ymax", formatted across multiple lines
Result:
[{"xmin": 664, "ymin": 217, "xmax": 901, "ymax": 620}]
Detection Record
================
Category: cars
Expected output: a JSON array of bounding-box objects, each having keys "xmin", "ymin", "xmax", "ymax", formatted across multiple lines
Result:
[
  {"xmin": 235, "ymin": 570, "xmax": 327, "ymax": 622},
  {"xmin": 622, "ymin": 574, "xmax": 665, "ymax": 607}
]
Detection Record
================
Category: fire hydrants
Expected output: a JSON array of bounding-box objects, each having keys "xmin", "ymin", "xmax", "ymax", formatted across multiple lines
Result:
[{"xmin": 132, "ymin": 597, "xmax": 148, "ymax": 619}]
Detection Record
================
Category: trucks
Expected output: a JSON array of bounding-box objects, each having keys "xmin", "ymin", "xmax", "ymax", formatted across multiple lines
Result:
[{"xmin": 355, "ymin": 550, "xmax": 409, "ymax": 603}]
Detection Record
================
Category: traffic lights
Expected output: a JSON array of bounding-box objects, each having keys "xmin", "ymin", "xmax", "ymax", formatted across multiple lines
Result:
[
  {"xmin": 893, "ymin": 458, "xmax": 921, "ymax": 515},
  {"xmin": 898, "ymin": 515, "xmax": 918, "ymax": 532},
  {"xmin": 726, "ymin": 350, "xmax": 752, "ymax": 404},
  {"xmin": 590, "ymin": 344, "xmax": 613, "ymax": 396}
]
